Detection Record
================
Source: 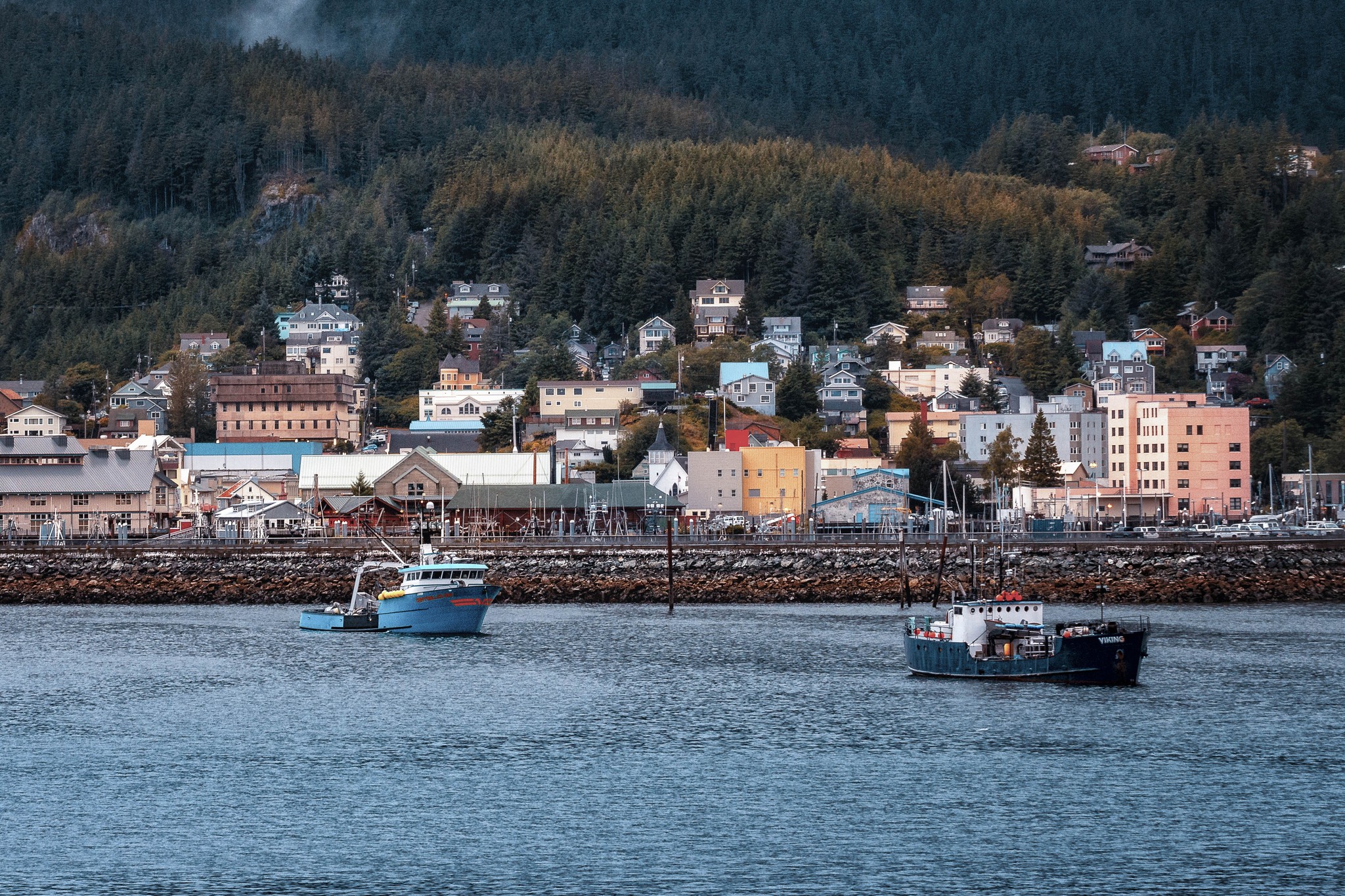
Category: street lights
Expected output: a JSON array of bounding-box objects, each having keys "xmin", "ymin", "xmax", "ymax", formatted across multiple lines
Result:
[
  {"xmin": 1107, "ymin": 502, "xmax": 1112, "ymax": 517},
  {"xmin": 92, "ymin": 380, "xmax": 97, "ymax": 421},
  {"xmin": 138, "ymin": 353, "xmax": 141, "ymax": 379},
  {"xmin": 144, "ymin": 355, "xmax": 150, "ymax": 376},
  {"xmin": 677, "ymin": 351, "xmax": 684, "ymax": 399},
  {"xmin": 1191, "ymin": 498, "xmax": 1195, "ymax": 523},
  {"xmin": 1341, "ymin": 480, "xmax": 1345, "ymax": 505},
  {"xmin": 1135, "ymin": 469, "xmax": 1147, "ymax": 526},
  {"xmin": 1258, "ymin": 481, "xmax": 1262, "ymax": 506},
  {"xmin": 261, "ymin": 326, "xmax": 265, "ymax": 362},
  {"xmin": 105, "ymin": 368, "xmax": 111, "ymax": 407},
  {"xmin": 51, "ymin": 506, "xmax": 61, "ymax": 546},
  {"xmin": 359, "ymin": 502, "xmax": 374, "ymax": 538},
  {"xmin": 717, "ymin": 502, "xmax": 723, "ymax": 535},
  {"xmin": 400, "ymin": 505, "xmax": 405, "ymax": 527},
  {"xmin": 1298, "ymin": 469, "xmax": 1311, "ymax": 520}
]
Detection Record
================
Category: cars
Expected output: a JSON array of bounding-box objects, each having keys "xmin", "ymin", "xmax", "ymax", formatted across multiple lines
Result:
[
  {"xmin": 1104, "ymin": 515, "xmax": 1345, "ymax": 537},
  {"xmin": 992, "ymin": 379, "xmax": 1014, "ymax": 414},
  {"xmin": 360, "ymin": 429, "xmax": 388, "ymax": 455},
  {"xmin": 405, "ymin": 299, "xmax": 420, "ymax": 323},
  {"xmin": 711, "ymin": 514, "xmax": 750, "ymax": 529},
  {"xmin": 597, "ymin": 362, "xmax": 610, "ymax": 381},
  {"xmin": 78, "ymin": 408, "xmax": 109, "ymax": 422},
  {"xmin": 1205, "ymin": 396, "xmax": 1239, "ymax": 407},
  {"xmin": 678, "ymin": 391, "xmax": 717, "ymax": 400}
]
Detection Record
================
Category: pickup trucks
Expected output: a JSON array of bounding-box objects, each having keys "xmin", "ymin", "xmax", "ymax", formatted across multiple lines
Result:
[{"xmin": 1244, "ymin": 398, "xmax": 1270, "ymax": 407}]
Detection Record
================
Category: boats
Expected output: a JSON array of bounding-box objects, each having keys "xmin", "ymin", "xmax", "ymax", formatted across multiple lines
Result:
[
  {"xmin": 903, "ymin": 469, "xmax": 1151, "ymax": 687},
  {"xmin": 299, "ymin": 492, "xmax": 503, "ymax": 634}
]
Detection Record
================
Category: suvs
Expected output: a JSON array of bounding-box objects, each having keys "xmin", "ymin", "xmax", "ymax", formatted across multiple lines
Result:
[{"xmin": 910, "ymin": 513, "xmax": 920, "ymax": 518}]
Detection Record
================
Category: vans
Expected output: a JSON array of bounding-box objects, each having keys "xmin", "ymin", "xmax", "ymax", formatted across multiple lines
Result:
[{"xmin": 946, "ymin": 511, "xmax": 954, "ymax": 521}]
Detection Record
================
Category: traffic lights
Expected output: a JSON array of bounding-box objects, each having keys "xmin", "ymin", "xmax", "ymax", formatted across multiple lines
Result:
[{"xmin": 821, "ymin": 492, "xmax": 828, "ymax": 501}]
